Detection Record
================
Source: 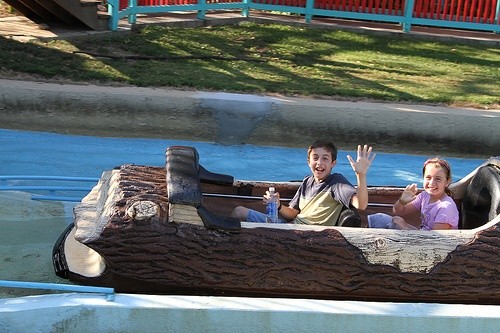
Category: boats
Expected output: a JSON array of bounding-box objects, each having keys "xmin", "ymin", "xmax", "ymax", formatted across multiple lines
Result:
[{"xmin": 51, "ymin": 145, "xmax": 500, "ymax": 305}]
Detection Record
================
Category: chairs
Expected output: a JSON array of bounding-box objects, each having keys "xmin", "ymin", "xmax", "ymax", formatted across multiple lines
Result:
[
  {"xmin": 459, "ymin": 166, "xmax": 500, "ymax": 229},
  {"xmin": 336, "ymin": 205, "xmax": 361, "ymax": 227}
]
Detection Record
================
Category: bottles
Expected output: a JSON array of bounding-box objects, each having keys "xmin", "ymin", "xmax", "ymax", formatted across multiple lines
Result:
[{"xmin": 266, "ymin": 187, "xmax": 278, "ymax": 224}]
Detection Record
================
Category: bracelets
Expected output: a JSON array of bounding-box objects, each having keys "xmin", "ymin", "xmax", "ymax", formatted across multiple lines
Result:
[{"xmin": 399, "ymin": 197, "xmax": 407, "ymax": 205}]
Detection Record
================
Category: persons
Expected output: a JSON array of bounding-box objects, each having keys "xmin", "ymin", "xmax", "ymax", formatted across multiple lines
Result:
[
  {"xmin": 229, "ymin": 140, "xmax": 376, "ymax": 226},
  {"xmin": 366, "ymin": 156, "xmax": 459, "ymax": 231}
]
